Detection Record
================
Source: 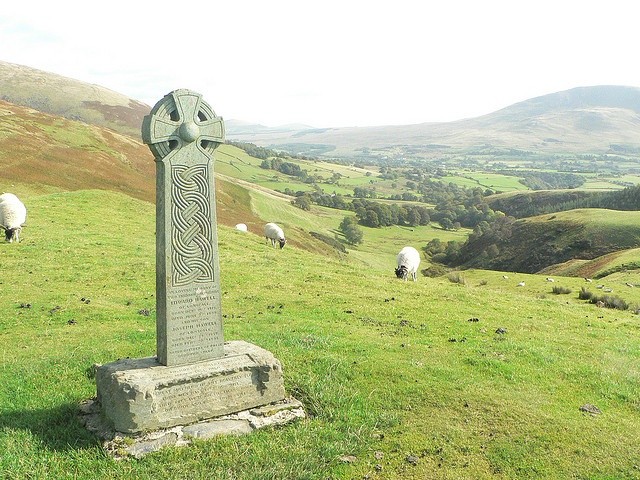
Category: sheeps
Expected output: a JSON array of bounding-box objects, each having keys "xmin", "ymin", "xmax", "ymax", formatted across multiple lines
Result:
[
  {"xmin": 394, "ymin": 246, "xmax": 421, "ymax": 282},
  {"xmin": 0, "ymin": 193, "xmax": 27, "ymax": 244},
  {"xmin": 264, "ymin": 222, "xmax": 287, "ymax": 250}
]
